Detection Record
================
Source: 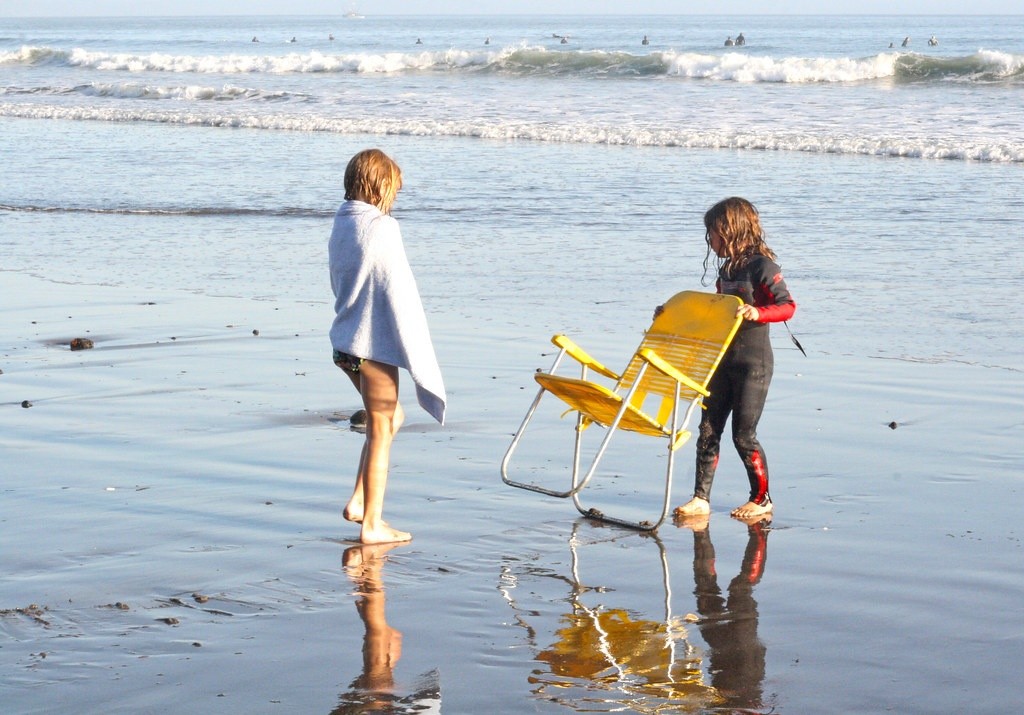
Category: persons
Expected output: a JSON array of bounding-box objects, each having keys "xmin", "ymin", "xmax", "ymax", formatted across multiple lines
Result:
[
  {"xmin": 415, "ymin": 37, "xmax": 423, "ymax": 44},
  {"xmin": 641, "ymin": 35, "xmax": 649, "ymax": 45},
  {"xmin": 901, "ymin": 37, "xmax": 910, "ymax": 47},
  {"xmin": 252, "ymin": 36, "xmax": 259, "ymax": 42},
  {"xmin": 652, "ymin": 195, "xmax": 795, "ymax": 518},
  {"xmin": 328, "ymin": 33, "xmax": 335, "ymax": 40},
  {"xmin": 670, "ymin": 513, "xmax": 774, "ymax": 714},
  {"xmin": 928, "ymin": 36, "xmax": 939, "ymax": 46},
  {"xmin": 553, "ymin": 34, "xmax": 561, "ymax": 38},
  {"xmin": 736, "ymin": 33, "xmax": 746, "ymax": 46},
  {"xmin": 561, "ymin": 37, "xmax": 569, "ymax": 44},
  {"xmin": 340, "ymin": 540, "xmax": 403, "ymax": 714},
  {"xmin": 724, "ymin": 36, "xmax": 735, "ymax": 46},
  {"xmin": 291, "ymin": 36, "xmax": 297, "ymax": 42},
  {"xmin": 484, "ymin": 37, "xmax": 491, "ymax": 45},
  {"xmin": 327, "ymin": 148, "xmax": 413, "ymax": 546},
  {"xmin": 888, "ymin": 43, "xmax": 895, "ymax": 48}
]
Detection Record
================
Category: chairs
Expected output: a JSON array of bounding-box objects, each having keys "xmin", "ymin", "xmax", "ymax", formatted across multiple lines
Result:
[{"xmin": 503, "ymin": 290, "xmax": 745, "ymax": 532}]
